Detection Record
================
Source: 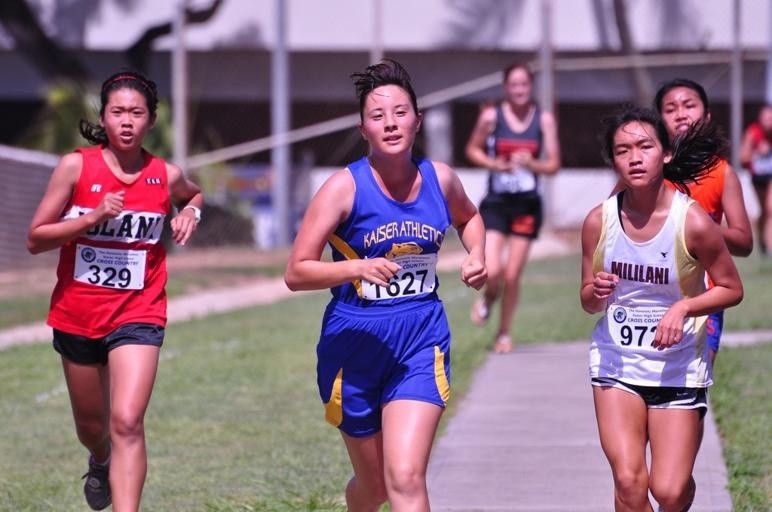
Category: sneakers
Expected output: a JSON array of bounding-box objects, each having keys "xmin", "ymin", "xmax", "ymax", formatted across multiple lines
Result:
[
  {"xmin": 83, "ymin": 440, "xmax": 112, "ymax": 510},
  {"xmin": 470, "ymin": 299, "xmax": 513, "ymax": 356}
]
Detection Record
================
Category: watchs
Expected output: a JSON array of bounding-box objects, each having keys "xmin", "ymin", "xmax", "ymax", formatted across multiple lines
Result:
[{"xmin": 183, "ymin": 205, "xmax": 203, "ymax": 225}]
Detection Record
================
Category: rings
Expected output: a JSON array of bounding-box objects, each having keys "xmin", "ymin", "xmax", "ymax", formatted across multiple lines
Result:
[{"xmin": 608, "ymin": 282, "xmax": 614, "ymax": 287}]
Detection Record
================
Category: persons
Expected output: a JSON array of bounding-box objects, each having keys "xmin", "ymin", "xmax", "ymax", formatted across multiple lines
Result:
[
  {"xmin": 736, "ymin": 100, "xmax": 772, "ymax": 262},
  {"xmin": 605, "ymin": 75, "xmax": 755, "ymax": 380},
  {"xmin": 23, "ymin": 66, "xmax": 206, "ymax": 512},
  {"xmin": 574, "ymin": 103, "xmax": 746, "ymax": 512},
  {"xmin": 462, "ymin": 54, "xmax": 562, "ymax": 358},
  {"xmin": 279, "ymin": 53, "xmax": 491, "ymax": 512}
]
